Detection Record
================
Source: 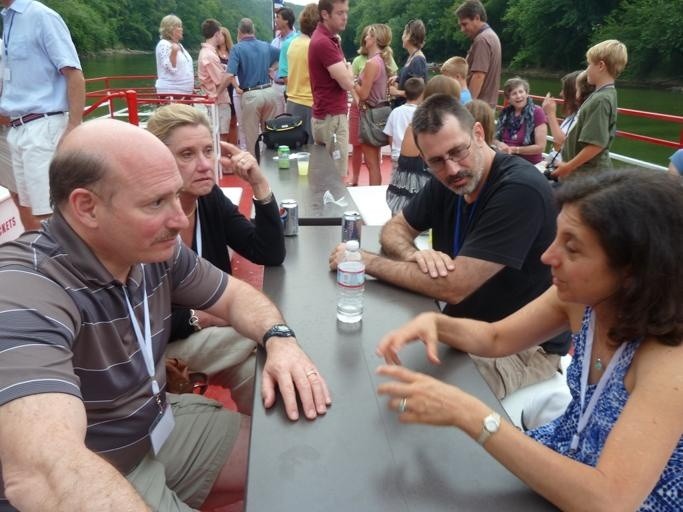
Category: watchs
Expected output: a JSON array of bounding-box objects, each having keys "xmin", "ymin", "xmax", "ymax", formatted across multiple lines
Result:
[
  {"xmin": 477, "ymin": 411, "xmax": 500, "ymax": 447},
  {"xmin": 263, "ymin": 324, "xmax": 295, "ymax": 348}
]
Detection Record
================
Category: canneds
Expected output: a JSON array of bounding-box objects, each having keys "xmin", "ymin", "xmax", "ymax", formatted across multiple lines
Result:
[
  {"xmin": 340, "ymin": 211, "xmax": 361, "ymax": 248},
  {"xmin": 277, "ymin": 145, "xmax": 290, "ymax": 168},
  {"xmin": 279, "ymin": 199, "xmax": 298, "ymax": 236}
]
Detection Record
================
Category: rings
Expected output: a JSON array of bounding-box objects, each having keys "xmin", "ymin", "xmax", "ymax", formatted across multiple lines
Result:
[
  {"xmin": 306, "ymin": 371, "xmax": 317, "ymax": 376},
  {"xmin": 399, "ymin": 399, "xmax": 406, "ymax": 409}
]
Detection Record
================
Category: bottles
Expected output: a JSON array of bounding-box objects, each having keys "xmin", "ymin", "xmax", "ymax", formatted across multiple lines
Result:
[{"xmin": 335, "ymin": 239, "xmax": 365, "ymax": 323}]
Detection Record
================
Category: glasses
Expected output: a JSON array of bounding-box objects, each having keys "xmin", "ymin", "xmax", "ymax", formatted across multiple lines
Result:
[
  {"xmin": 423, "ymin": 143, "xmax": 470, "ymax": 176},
  {"xmin": 407, "ymin": 18, "xmax": 416, "ymax": 30}
]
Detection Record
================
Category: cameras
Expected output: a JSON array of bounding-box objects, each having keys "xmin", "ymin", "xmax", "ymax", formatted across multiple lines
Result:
[{"xmin": 544, "ymin": 164, "xmax": 558, "ymax": 182}]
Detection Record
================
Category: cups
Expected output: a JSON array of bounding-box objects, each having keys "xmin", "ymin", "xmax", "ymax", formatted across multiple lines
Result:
[{"xmin": 294, "ymin": 151, "xmax": 311, "ymax": 176}]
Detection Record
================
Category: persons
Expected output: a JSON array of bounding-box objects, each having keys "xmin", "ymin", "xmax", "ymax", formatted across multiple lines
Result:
[
  {"xmin": 377, "ymin": 167, "xmax": 683, "ymax": 512},
  {"xmin": 154, "ymin": 1, "xmax": 627, "ymax": 226},
  {"xmin": 329, "ymin": 94, "xmax": 569, "ymax": 404},
  {"xmin": 1, "ymin": 0, "xmax": 85, "ymax": 237},
  {"xmin": 0, "ymin": 120, "xmax": 331, "ymax": 512},
  {"xmin": 150, "ymin": 104, "xmax": 286, "ymax": 414},
  {"xmin": 669, "ymin": 149, "xmax": 683, "ymax": 183}
]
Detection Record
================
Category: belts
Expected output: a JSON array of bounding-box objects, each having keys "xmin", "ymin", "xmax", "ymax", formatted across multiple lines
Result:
[
  {"xmin": 9, "ymin": 111, "xmax": 62, "ymax": 126},
  {"xmin": 243, "ymin": 84, "xmax": 270, "ymax": 92}
]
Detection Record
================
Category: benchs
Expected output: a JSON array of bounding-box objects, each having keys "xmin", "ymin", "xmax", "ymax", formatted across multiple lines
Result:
[
  {"xmin": 502, "ymin": 354, "xmax": 572, "ymax": 428},
  {"xmin": 346, "ymin": 186, "xmax": 429, "ymax": 249},
  {"xmin": 216, "ymin": 188, "xmax": 244, "ymax": 260}
]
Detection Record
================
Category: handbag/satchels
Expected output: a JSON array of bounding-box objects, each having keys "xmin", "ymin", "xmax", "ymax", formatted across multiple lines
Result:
[
  {"xmin": 358, "ymin": 105, "xmax": 392, "ymax": 148},
  {"xmin": 254, "ymin": 113, "xmax": 307, "ymax": 167},
  {"xmin": 165, "ymin": 356, "xmax": 207, "ymax": 395}
]
